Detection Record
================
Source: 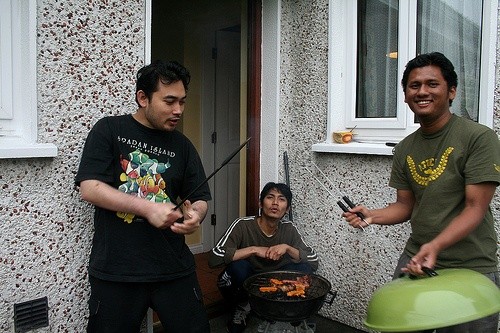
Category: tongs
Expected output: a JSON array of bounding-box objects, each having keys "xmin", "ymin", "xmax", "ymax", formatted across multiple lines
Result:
[{"xmin": 294, "ymin": 149, "xmax": 374, "ymax": 235}]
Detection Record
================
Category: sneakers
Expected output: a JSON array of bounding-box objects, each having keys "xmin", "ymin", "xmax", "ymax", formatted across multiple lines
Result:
[{"xmin": 230, "ymin": 306, "xmax": 248, "ymax": 332}]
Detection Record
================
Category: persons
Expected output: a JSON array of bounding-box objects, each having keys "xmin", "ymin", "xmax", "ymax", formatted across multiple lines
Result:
[
  {"xmin": 341, "ymin": 51, "xmax": 500, "ymax": 333},
  {"xmin": 75, "ymin": 59, "xmax": 211, "ymax": 333},
  {"xmin": 208, "ymin": 182, "xmax": 318, "ymax": 333}
]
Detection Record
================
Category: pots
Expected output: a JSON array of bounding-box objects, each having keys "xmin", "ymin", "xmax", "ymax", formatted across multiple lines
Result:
[{"xmin": 242, "ymin": 270, "xmax": 337, "ymax": 321}]
